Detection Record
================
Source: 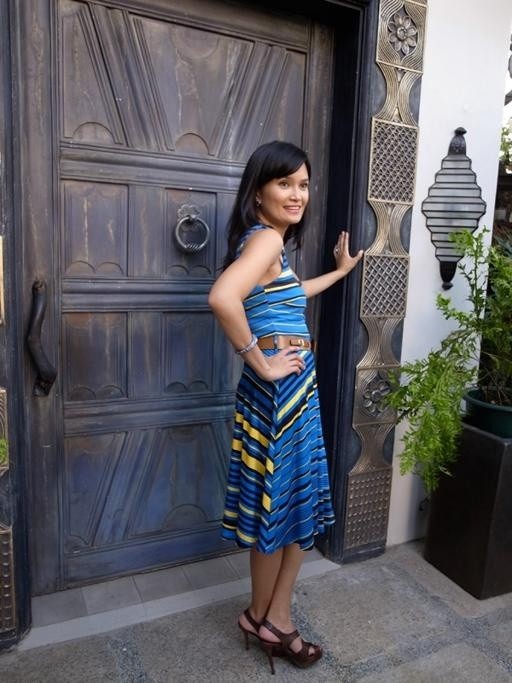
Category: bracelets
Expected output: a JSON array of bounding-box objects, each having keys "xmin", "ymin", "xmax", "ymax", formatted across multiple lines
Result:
[{"xmin": 234, "ymin": 336, "xmax": 258, "ymax": 356}]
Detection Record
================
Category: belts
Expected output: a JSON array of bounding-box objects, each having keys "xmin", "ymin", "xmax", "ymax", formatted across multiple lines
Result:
[{"xmin": 255, "ymin": 334, "xmax": 310, "ymax": 351}]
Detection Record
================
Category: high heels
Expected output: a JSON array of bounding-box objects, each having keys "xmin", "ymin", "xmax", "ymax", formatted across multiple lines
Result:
[{"xmin": 238, "ymin": 608, "xmax": 322, "ymax": 674}]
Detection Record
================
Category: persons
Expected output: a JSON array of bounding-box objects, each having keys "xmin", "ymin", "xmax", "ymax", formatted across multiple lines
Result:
[{"xmin": 205, "ymin": 139, "xmax": 365, "ymax": 675}]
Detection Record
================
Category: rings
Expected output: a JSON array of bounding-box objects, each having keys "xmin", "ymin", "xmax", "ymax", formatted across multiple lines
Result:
[{"xmin": 335, "ymin": 248, "xmax": 339, "ymax": 254}]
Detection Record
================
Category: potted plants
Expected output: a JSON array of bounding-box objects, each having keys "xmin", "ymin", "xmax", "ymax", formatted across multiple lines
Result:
[{"xmin": 383, "ymin": 226, "xmax": 511, "ymax": 493}]
